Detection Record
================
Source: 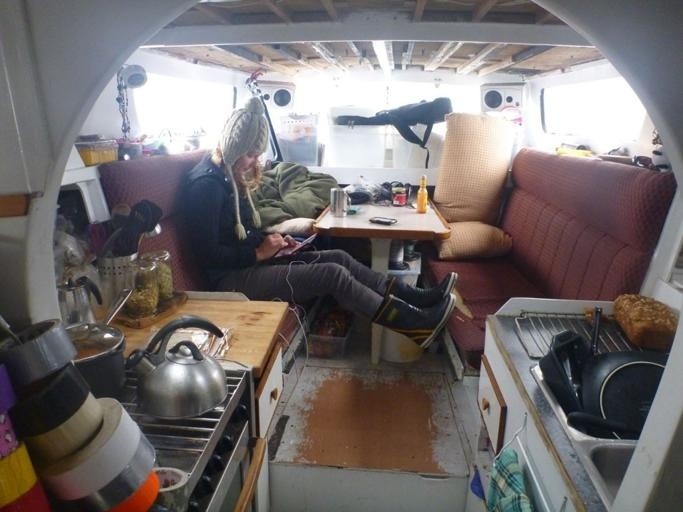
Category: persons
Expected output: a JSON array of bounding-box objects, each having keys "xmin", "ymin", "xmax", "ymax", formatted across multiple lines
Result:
[{"xmin": 176, "ymin": 98, "xmax": 458, "ymax": 350}]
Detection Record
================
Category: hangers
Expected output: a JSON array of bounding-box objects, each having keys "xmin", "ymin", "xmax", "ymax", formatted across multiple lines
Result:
[{"xmin": 491, "ymin": 412, "xmax": 550, "ymax": 512}]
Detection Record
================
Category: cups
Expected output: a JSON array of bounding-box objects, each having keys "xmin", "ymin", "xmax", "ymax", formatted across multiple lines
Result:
[{"xmin": 330, "ymin": 189, "xmax": 351, "ymax": 218}]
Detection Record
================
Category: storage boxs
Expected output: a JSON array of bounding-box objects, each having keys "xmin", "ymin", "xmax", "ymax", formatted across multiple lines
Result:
[
  {"xmin": 305, "ymin": 316, "xmax": 357, "ymax": 359},
  {"xmin": 74, "ymin": 140, "xmax": 119, "ymax": 167}
]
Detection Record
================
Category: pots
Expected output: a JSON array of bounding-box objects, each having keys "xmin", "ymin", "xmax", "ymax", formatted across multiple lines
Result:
[{"xmin": 65, "ymin": 290, "xmax": 133, "ymax": 365}]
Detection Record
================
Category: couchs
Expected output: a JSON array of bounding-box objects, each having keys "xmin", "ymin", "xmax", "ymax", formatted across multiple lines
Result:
[
  {"xmin": 97, "ymin": 148, "xmax": 322, "ymax": 372},
  {"xmin": 416, "ymin": 145, "xmax": 676, "ymax": 381}
]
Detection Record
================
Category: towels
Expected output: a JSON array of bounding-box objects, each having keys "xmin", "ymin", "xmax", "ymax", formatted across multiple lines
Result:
[{"xmin": 486, "ymin": 446, "xmax": 535, "ymax": 512}]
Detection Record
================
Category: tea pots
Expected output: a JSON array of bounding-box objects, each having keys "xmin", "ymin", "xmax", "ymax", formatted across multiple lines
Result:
[
  {"xmin": 123, "ymin": 315, "xmax": 227, "ymax": 420},
  {"xmin": 58, "ymin": 276, "xmax": 102, "ymax": 330}
]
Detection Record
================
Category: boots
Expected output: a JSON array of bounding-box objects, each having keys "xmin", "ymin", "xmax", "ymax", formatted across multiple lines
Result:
[
  {"xmin": 369, "ymin": 292, "xmax": 457, "ymax": 350},
  {"xmin": 384, "ymin": 270, "xmax": 459, "ymax": 306}
]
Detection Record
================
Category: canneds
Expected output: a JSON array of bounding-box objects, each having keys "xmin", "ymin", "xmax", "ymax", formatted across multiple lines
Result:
[{"xmin": 392, "ymin": 187, "xmax": 407, "ymax": 207}]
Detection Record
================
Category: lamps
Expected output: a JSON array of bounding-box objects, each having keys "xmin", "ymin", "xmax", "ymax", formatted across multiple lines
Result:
[{"xmin": 116, "ymin": 61, "xmax": 147, "ymax": 159}]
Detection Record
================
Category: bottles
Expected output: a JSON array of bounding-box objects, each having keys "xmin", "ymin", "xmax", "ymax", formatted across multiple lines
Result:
[
  {"xmin": 123, "ymin": 248, "xmax": 175, "ymax": 319},
  {"xmin": 416, "ymin": 175, "xmax": 427, "ymax": 213}
]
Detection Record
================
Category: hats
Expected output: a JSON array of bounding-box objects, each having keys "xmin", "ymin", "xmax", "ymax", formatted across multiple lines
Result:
[{"xmin": 217, "ymin": 97, "xmax": 271, "ymax": 171}]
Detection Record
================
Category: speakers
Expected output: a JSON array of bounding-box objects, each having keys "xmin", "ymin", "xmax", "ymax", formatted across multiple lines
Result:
[
  {"xmin": 480, "ymin": 82, "xmax": 526, "ymax": 112},
  {"xmin": 256, "ymin": 80, "xmax": 295, "ymax": 110}
]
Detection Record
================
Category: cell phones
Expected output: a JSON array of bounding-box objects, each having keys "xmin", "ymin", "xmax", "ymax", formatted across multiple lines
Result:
[{"xmin": 370, "ymin": 217, "xmax": 397, "ymax": 224}]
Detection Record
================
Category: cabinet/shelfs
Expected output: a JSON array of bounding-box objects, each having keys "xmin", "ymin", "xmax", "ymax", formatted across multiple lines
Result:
[
  {"xmin": 465, "ymin": 322, "xmax": 577, "ymax": 512},
  {"xmin": 249, "ymin": 341, "xmax": 284, "ymax": 512}
]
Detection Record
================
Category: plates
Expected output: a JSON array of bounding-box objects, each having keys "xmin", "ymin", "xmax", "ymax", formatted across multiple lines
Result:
[{"xmin": 542, "ymin": 303, "xmax": 668, "ymax": 440}]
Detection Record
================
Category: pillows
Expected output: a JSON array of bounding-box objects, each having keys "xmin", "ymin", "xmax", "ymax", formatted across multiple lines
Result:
[
  {"xmin": 432, "ymin": 219, "xmax": 513, "ymax": 261},
  {"xmin": 431, "ymin": 111, "xmax": 514, "ymax": 223}
]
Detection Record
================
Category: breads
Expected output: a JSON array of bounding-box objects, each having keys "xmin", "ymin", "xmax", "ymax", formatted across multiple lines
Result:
[{"xmin": 614, "ymin": 293, "xmax": 678, "ymax": 351}]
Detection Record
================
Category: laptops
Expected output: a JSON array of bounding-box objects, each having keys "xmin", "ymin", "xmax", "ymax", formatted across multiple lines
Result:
[{"xmin": 273, "ymin": 233, "xmax": 317, "ymax": 258}]
